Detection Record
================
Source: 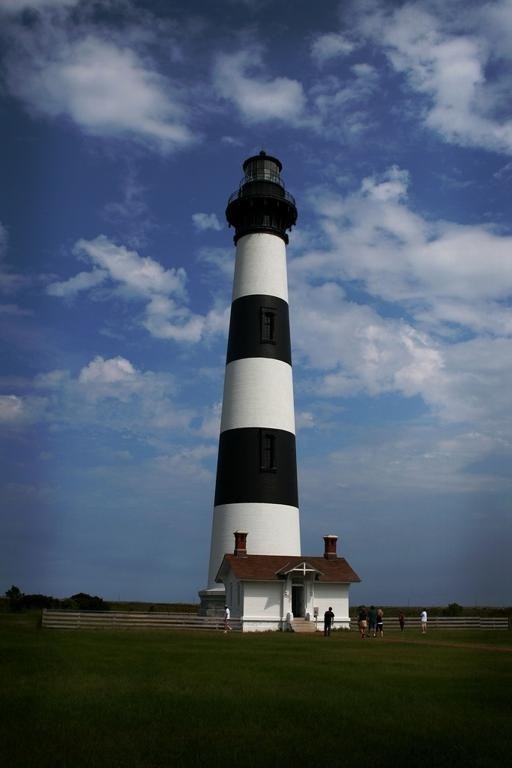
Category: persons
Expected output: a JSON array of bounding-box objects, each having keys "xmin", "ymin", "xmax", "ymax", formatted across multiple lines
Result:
[
  {"xmin": 376, "ymin": 608, "xmax": 384, "ymax": 638},
  {"xmin": 398, "ymin": 610, "xmax": 405, "ymax": 632},
  {"xmin": 223, "ymin": 604, "xmax": 232, "ymax": 634},
  {"xmin": 367, "ymin": 605, "xmax": 377, "ymax": 639},
  {"xmin": 420, "ymin": 608, "xmax": 427, "ymax": 634},
  {"xmin": 323, "ymin": 606, "xmax": 335, "ymax": 637},
  {"xmin": 358, "ymin": 608, "xmax": 367, "ymax": 639}
]
